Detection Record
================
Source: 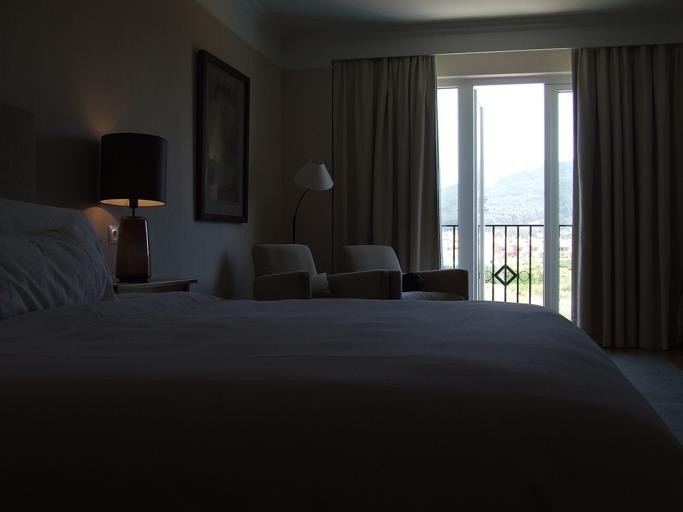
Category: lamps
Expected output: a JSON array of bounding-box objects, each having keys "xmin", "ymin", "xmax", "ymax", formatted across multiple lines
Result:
[
  {"xmin": 96, "ymin": 133, "xmax": 168, "ymax": 284},
  {"xmin": 293, "ymin": 160, "xmax": 335, "ymax": 244}
]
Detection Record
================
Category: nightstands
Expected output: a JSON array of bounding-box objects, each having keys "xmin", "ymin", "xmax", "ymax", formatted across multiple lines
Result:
[{"xmin": 111, "ymin": 278, "xmax": 198, "ymax": 294}]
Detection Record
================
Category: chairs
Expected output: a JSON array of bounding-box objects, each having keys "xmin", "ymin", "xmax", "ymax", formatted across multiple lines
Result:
[
  {"xmin": 341, "ymin": 245, "xmax": 469, "ymax": 301},
  {"xmin": 252, "ymin": 242, "xmax": 390, "ymax": 300}
]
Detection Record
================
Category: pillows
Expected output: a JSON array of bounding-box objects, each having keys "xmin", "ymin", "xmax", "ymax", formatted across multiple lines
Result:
[{"xmin": 0, "ymin": 197, "xmax": 118, "ymax": 320}]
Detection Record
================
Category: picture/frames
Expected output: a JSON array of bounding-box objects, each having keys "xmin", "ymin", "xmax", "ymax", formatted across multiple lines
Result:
[{"xmin": 196, "ymin": 47, "xmax": 251, "ymax": 224}]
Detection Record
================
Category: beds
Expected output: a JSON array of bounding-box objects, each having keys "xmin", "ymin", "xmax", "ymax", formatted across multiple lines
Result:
[{"xmin": 0, "ymin": 101, "xmax": 683, "ymax": 512}]
